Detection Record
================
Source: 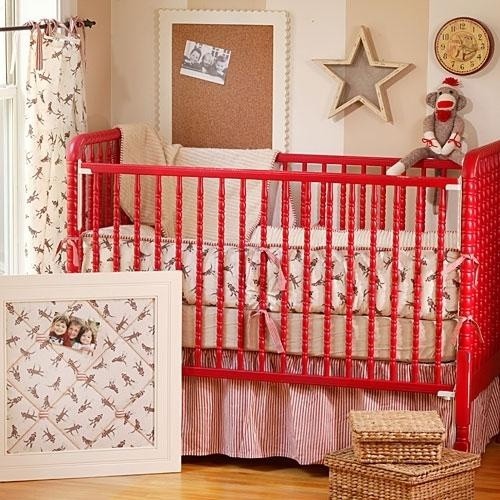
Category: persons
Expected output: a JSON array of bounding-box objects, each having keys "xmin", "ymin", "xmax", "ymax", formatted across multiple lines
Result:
[
  {"xmin": 182, "ymin": 48, "xmax": 227, "ymax": 80},
  {"xmin": 49, "ymin": 316, "xmax": 95, "ymax": 356}
]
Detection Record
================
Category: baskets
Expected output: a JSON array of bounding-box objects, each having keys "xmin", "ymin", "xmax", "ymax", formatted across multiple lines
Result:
[
  {"xmin": 324, "ymin": 446, "xmax": 481, "ymax": 500},
  {"xmin": 348, "ymin": 409, "xmax": 445, "ymax": 464}
]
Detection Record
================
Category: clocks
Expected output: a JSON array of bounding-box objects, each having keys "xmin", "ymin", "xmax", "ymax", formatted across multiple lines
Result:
[{"xmin": 434, "ymin": 15, "xmax": 495, "ymax": 77}]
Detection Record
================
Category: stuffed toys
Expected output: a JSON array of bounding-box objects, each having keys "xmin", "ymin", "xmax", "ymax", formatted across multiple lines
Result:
[{"xmin": 385, "ymin": 77, "xmax": 467, "ymax": 175}]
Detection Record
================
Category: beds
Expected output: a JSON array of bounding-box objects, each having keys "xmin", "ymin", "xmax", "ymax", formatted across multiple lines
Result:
[{"xmin": 64, "ymin": 127, "xmax": 500, "ymax": 453}]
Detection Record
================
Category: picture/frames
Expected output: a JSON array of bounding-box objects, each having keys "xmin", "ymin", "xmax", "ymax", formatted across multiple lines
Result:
[
  {"xmin": 158, "ymin": 10, "xmax": 291, "ymax": 152},
  {"xmin": 0, "ymin": 270, "xmax": 182, "ymax": 484}
]
